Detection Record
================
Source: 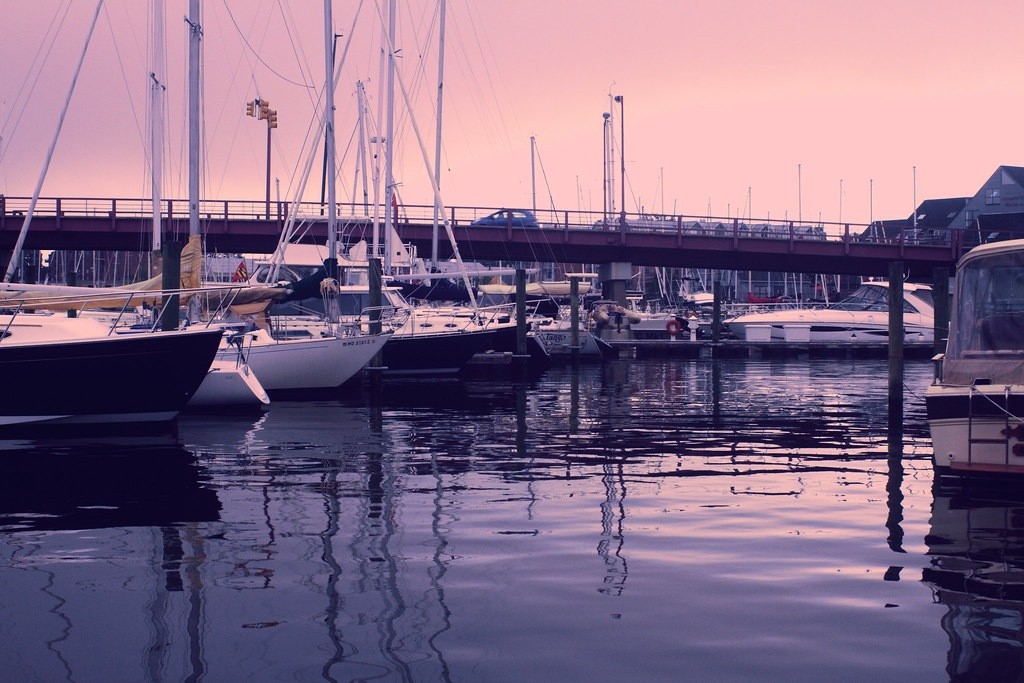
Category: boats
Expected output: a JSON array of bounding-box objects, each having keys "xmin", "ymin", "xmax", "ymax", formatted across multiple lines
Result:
[{"xmin": 0, "ymin": 0, "xmax": 1024, "ymax": 476}]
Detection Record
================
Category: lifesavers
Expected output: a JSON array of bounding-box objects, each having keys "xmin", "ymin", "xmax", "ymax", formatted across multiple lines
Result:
[{"xmin": 666, "ymin": 320, "xmax": 680, "ymax": 334}]
